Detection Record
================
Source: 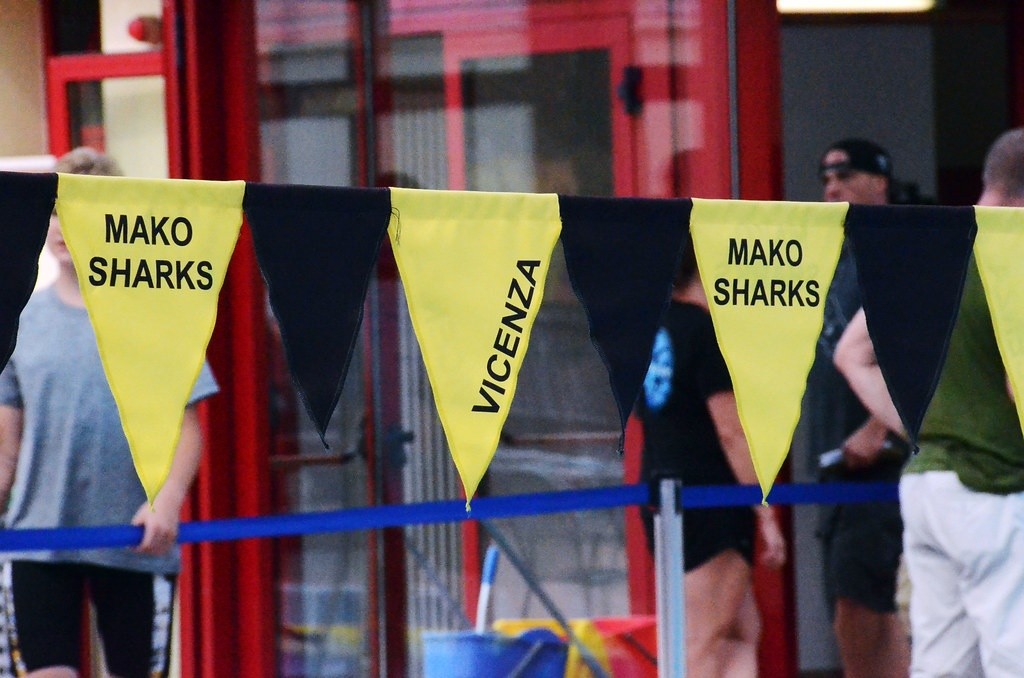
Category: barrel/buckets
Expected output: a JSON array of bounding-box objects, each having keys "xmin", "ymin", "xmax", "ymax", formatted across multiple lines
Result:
[{"xmin": 421, "ymin": 612, "xmax": 657, "ymax": 678}]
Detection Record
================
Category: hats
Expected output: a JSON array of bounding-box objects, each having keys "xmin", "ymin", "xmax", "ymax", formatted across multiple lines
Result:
[{"xmin": 819, "ymin": 139, "xmax": 894, "ymax": 180}]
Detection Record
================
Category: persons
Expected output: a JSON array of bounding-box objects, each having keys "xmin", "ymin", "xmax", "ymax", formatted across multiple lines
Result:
[
  {"xmin": 632, "ymin": 239, "xmax": 788, "ymax": 677},
  {"xmin": 808, "ymin": 138, "xmax": 913, "ymax": 678},
  {"xmin": 1, "ymin": 148, "xmax": 221, "ymax": 678},
  {"xmin": 831, "ymin": 127, "xmax": 1024, "ymax": 678}
]
